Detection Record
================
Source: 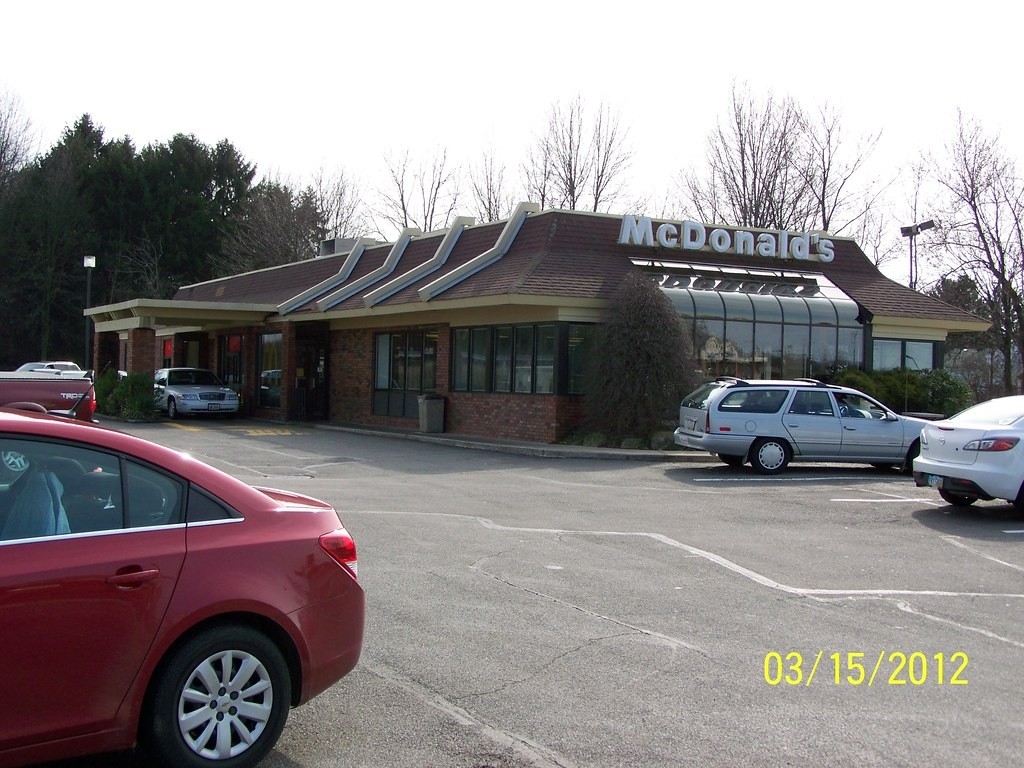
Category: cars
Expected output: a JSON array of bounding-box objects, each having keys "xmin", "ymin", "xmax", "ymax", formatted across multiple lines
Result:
[
  {"xmin": 0, "ymin": 402, "xmax": 366, "ymax": 768},
  {"xmin": 14, "ymin": 360, "xmax": 94, "ymax": 382},
  {"xmin": 155, "ymin": 365, "xmax": 241, "ymax": 420},
  {"xmin": 261, "ymin": 368, "xmax": 282, "ymax": 378},
  {"xmin": 911, "ymin": 394, "xmax": 1024, "ymax": 520}
]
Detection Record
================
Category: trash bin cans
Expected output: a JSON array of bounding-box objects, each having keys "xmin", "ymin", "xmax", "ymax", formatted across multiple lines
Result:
[{"xmin": 416, "ymin": 391, "xmax": 448, "ymax": 433}]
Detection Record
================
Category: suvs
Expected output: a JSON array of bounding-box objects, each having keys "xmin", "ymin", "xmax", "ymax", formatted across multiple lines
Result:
[{"xmin": 673, "ymin": 375, "xmax": 931, "ymax": 476}]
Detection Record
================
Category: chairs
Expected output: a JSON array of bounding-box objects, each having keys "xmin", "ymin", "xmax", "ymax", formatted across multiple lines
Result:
[{"xmin": 177, "ymin": 374, "xmax": 193, "ymax": 385}]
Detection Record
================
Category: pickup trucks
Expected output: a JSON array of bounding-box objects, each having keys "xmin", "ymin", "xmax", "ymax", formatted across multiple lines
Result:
[{"xmin": 0, "ymin": 370, "xmax": 100, "ymax": 480}]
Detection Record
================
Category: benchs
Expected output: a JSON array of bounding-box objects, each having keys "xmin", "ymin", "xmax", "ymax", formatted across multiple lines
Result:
[{"xmin": 47, "ymin": 456, "xmax": 166, "ymax": 536}]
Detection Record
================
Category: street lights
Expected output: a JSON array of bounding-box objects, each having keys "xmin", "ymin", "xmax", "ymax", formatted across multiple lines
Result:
[
  {"xmin": 900, "ymin": 220, "xmax": 936, "ymax": 290},
  {"xmin": 83, "ymin": 255, "xmax": 95, "ymax": 370}
]
